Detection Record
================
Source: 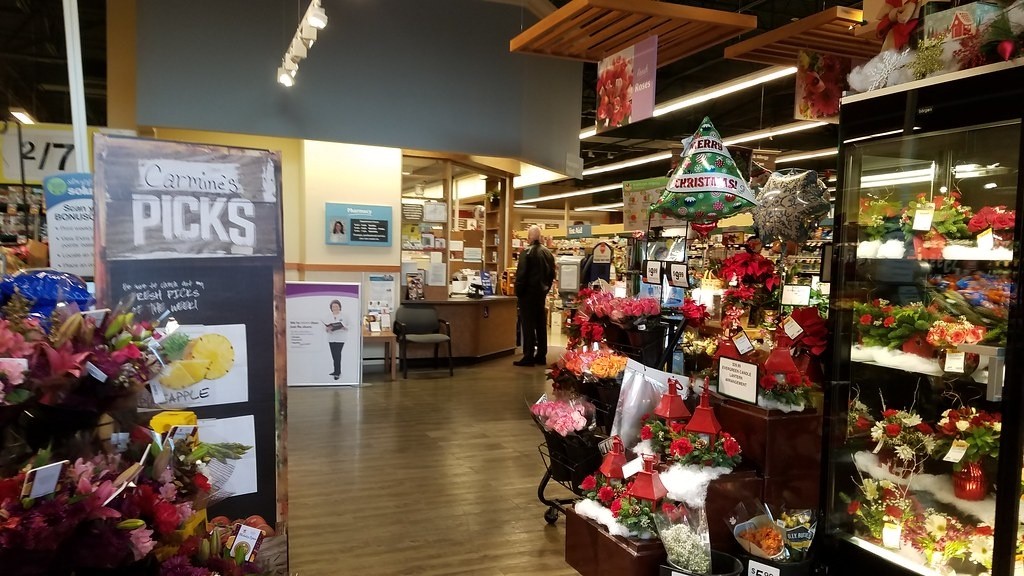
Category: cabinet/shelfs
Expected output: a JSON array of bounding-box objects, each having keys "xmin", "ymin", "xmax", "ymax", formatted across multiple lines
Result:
[
  {"xmin": 400, "ymin": 156, "xmax": 452, "ymax": 301},
  {"xmin": 483, "ymin": 177, "xmax": 513, "ymax": 286},
  {"xmin": 513, "ymin": 214, "xmax": 833, "ymax": 298},
  {"xmin": 817, "ymin": 57, "xmax": 1024, "ymax": 576}
]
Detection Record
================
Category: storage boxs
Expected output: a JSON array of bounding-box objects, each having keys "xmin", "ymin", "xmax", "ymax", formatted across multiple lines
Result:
[
  {"xmin": 402, "ymin": 251, "xmax": 447, "ymax": 286},
  {"xmin": 924, "ymin": 2, "xmax": 1005, "ymax": 46},
  {"xmin": 421, "ymin": 232, "xmax": 435, "ymax": 249},
  {"xmin": 434, "ymin": 236, "xmax": 446, "ymax": 249}
]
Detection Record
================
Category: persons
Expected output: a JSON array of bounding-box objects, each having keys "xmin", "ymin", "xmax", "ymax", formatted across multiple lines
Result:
[
  {"xmin": 514, "ymin": 226, "xmax": 555, "ymax": 366},
  {"xmin": 325, "ymin": 300, "xmax": 348, "ymax": 380},
  {"xmin": 331, "ymin": 221, "xmax": 345, "ymax": 242}
]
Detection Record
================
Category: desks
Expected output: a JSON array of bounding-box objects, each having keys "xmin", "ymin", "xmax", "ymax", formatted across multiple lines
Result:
[{"xmin": 363, "ymin": 330, "xmax": 398, "ymax": 381}]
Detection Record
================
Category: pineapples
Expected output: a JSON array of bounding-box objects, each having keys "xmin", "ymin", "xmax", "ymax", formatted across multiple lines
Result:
[{"xmin": 159, "ymin": 334, "xmax": 235, "ymax": 389}]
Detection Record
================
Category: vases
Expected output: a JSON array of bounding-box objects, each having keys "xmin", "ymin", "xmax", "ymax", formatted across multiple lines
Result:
[
  {"xmin": 936, "ymin": 348, "xmax": 980, "ymax": 376},
  {"xmin": 952, "ymin": 457, "xmax": 989, "ymax": 502},
  {"xmin": 912, "ymin": 228, "xmax": 947, "ymax": 258},
  {"xmin": 901, "ymin": 331, "xmax": 938, "ymax": 359}
]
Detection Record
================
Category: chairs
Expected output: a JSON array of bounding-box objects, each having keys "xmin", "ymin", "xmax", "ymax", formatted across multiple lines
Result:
[{"xmin": 393, "ymin": 305, "xmax": 453, "ymax": 379}]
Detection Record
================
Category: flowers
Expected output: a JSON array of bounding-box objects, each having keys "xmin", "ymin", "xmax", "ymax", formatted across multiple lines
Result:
[
  {"xmin": 531, "ymin": 191, "xmax": 1024, "ymax": 576},
  {"xmin": 0, "ymin": 286, "xmax": 254, "ymax": 576}
]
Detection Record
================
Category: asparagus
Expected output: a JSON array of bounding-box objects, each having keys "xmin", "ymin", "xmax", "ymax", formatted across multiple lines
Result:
[{"xmin": 198, "ymin": 441, "xmax": 254, "ymax": 464}]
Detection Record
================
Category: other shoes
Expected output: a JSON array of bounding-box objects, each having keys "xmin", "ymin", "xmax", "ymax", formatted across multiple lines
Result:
[
  {"xmin": 534, "ymin": 354, "xmax": 546, "ymax": 366},
  {"xmin": 512, "ymin": 355, "xmax": 535, "ymax": 367},
  {"xmin": 329, "ymin": 370, "xmax": 341, "ymax": 375},
  {"xmin": 334, "ymin": 372, "xmax": 339, "ymax": 380}
]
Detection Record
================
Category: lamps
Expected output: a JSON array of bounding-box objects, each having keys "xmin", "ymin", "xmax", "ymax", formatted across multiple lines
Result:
[
  {"xmin": 276, "ymin": 0, "xmax": 329, "ymax": 93},
  {"xmin": 8, "ymin": 79, "xmax": 38, "ymax": 125}
]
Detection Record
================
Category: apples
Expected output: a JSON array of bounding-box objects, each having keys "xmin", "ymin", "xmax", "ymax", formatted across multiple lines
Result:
[{"xmin": 205, "ymin": 515, "xmax": 275, "ymax": 542}]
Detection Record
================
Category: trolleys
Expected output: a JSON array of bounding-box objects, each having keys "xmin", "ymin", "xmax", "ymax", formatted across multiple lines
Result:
[{"xmin": 536, "ymin": 418, "xmax": 607, "ymax": 523}]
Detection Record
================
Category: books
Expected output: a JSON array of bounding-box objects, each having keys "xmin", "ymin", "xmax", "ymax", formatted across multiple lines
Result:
[{"xmin": 321, "ymin": 320, "xmax": 344, "ymax": 331}]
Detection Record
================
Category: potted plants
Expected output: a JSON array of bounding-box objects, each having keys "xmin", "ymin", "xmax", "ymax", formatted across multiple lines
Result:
[{"xmin": 483, "ymin": 190, "xmax": 500, "ymax": 209}]
